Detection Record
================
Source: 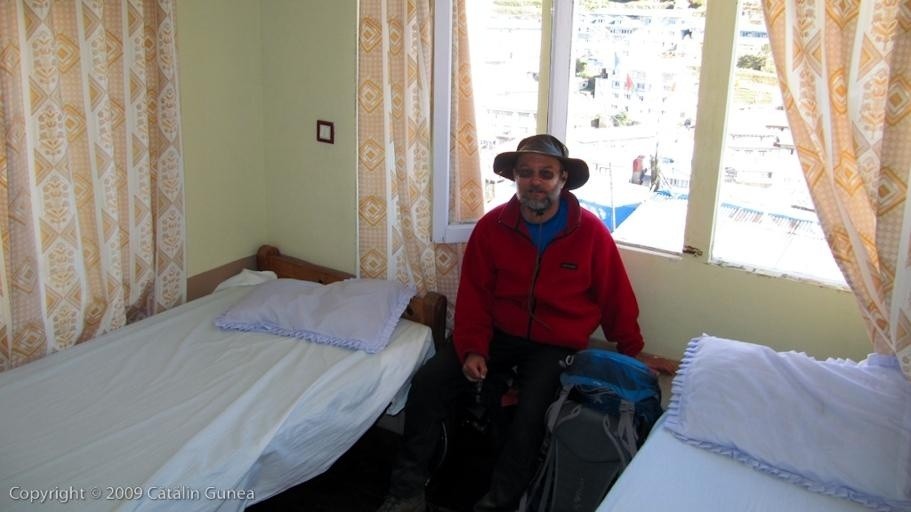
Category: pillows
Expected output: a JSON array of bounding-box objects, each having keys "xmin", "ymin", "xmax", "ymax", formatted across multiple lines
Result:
[
  {"xmin": 212, "ymin": 277, "xmax": 416, "ymax": 354},
  {"xmin": 661, "ymin": 330, "xmax": 908, "ymax": 512}
]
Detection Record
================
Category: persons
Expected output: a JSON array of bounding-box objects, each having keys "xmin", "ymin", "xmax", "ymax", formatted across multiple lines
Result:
[{"xmin": 389, "ymin": 131, "xmax": 684, "ymax": 510}]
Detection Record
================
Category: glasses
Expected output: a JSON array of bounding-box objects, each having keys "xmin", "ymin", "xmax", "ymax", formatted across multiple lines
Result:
[{"xmin": 515, "ymin": 166, "xmax": 563, "ymax": 181}]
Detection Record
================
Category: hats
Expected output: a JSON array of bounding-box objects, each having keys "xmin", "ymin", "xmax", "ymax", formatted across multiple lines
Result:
[{"xmin": 493, "ymin": 134, "xmax": 590, "ymax": 192}]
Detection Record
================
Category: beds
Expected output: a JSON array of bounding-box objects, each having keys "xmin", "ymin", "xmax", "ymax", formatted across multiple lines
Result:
[
  {"xmin": 0, "ymin": 245, "xmax": 447, "ymax": 511},
  {"xmin": 594, "ymin": 410, "xmax": 910, "ymax": 510}
]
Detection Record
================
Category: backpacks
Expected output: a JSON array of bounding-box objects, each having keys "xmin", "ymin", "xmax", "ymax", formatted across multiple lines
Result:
[{"xmin": 518, "ymin": 349, "xmax": 664, "ymax": 512}]
